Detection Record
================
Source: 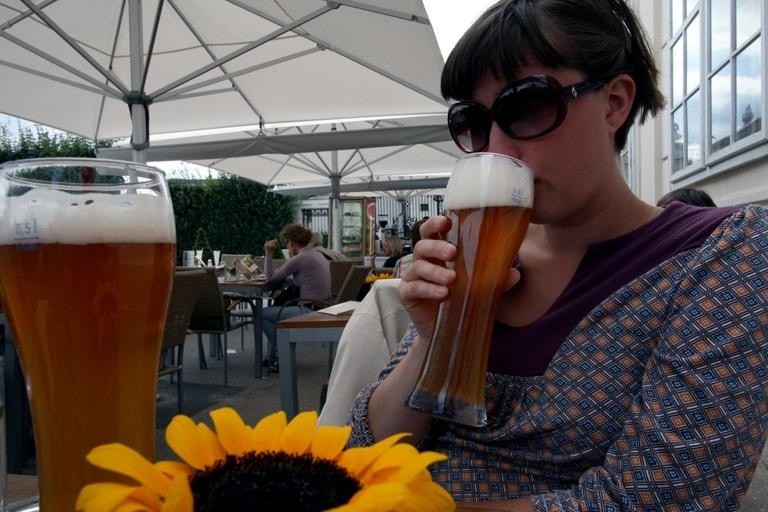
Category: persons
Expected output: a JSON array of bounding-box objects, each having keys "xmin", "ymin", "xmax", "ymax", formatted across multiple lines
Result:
[
  {"xmin": 655, "ymin": 187, "xmax": 720, "ymax": 209},
  {"xmin": 273, "ymin": 229, "xmax": 352, "ymax": 307},
  {"xmin": 261, "ymin": 224, "xmax": 331, "ymax": 372},
  {"xmin": 383, "ymin": 237, "xmax": 404, "ymax": 268},
  {"xmin": 344, "ymin": 0, "xmax": 768, "ymax": 512},
  {"xmin": 392, "ymin": 218, "xmax": 453, "ymax": 278}
]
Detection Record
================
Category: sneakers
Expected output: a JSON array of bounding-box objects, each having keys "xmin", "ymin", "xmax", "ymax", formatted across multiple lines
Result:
[{"xmin": 262, "ymin": 357, "xmax": 279, "ymax": 375}]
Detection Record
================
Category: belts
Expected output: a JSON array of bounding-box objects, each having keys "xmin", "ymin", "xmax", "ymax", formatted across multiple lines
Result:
[{"xmin": 303, "ymin": 303, "xmax": 321, "ymax": 311}]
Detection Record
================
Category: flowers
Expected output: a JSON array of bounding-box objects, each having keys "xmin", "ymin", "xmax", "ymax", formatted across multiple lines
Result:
[{"xmin": 75, "ymin": 403, "xmax": 461, "ymax": 512}]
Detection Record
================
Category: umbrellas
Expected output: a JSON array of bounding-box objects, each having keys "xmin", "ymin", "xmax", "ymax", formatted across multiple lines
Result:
[
  {"xmin": 0, "ymin": 0, "xmax": 454, "ymax": 166},
  {"xmin": 267, "ymin": 175, "xmax": 453, "ymax": 219},
  {"xmin": 159, "ymin": 114, "xmax": 471, "ymax": 254}
]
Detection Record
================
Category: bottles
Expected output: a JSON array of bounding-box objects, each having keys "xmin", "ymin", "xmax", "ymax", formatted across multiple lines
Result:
[{"xmin": 207, "ymin": 258, "xmax": 212, "ymax": 267}]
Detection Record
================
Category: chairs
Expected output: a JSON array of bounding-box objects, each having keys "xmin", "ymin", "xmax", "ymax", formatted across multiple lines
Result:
[{"xmin": 151, "ymin": 264, "xmax": 373, "ymax": 425}]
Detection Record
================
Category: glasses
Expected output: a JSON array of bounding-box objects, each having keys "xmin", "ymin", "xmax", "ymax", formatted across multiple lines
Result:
[{"xmin": 447, "ymin": 73, "xmax": 605, "ymax": 153}]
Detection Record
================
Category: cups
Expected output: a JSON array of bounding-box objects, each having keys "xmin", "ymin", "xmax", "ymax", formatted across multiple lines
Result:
[
  {"xmin": 196, "ymin": 249, "xmax": 203, "ymax": 267},
  {"xmin": 213, "ymin": 249, "xmax": 221, "ymax": 266},
  {"xmin": 404, "ymin": 149, "xmax": 536, "ymax": 430},
  {"xmin": 0, "ymin": 151, "xmax": 180, "ymax": 512}
]
